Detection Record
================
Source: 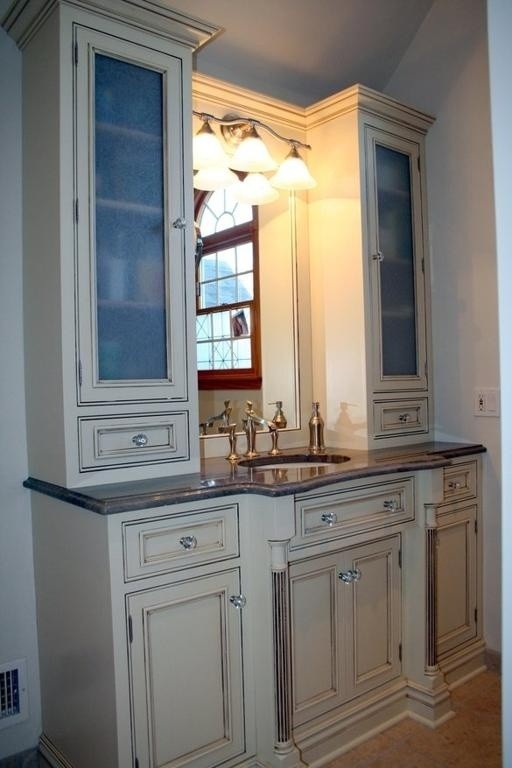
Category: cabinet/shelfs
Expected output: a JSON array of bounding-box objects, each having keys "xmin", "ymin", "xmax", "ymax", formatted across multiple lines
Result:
[
  {"xmin": 3, "ymin": 0, "xmax": 226, "ymax": 487},
  {"xmin": 260, "ymin": 465, "xmax": 458, "ymax": 766},
  {"xmin": 423, "ymin": 452, "xmax": 490, "ymax": 726},
  {"xmin": 305, "ymin": 82, "xmax": 437, "ymax": 454},
  {"xmin": 12, "ymin": 474, "xmax": 264, "ymax": 766}
]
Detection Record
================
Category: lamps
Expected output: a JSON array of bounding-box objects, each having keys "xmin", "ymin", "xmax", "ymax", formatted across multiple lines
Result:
[
  {"xmin": 192, "ymin": 165, "xmax": 277, "ymax": 203},
  {"xmin": 191, "ymin": 107, "xmax": 321, "ymax": 193}
]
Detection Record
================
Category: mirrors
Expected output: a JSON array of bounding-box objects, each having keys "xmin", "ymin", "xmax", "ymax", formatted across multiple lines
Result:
[{"xmin": 192, "ymin": 149, "xmax": 302, "ymax": 441}]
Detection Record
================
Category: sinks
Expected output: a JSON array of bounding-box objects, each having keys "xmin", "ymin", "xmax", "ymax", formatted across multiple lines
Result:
[{"xmin": 237, "ymin": 452, "xmax": 352, "ymax": 470}]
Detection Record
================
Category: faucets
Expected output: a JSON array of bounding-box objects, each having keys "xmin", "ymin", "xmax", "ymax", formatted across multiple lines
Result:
[
  {"xmin": 206, "ymin": 407, "xmax": 233, "ymax": 428},
  {"xmin": 242, "ymin": 410, "xmax": 278, "ymax": 457}
]
